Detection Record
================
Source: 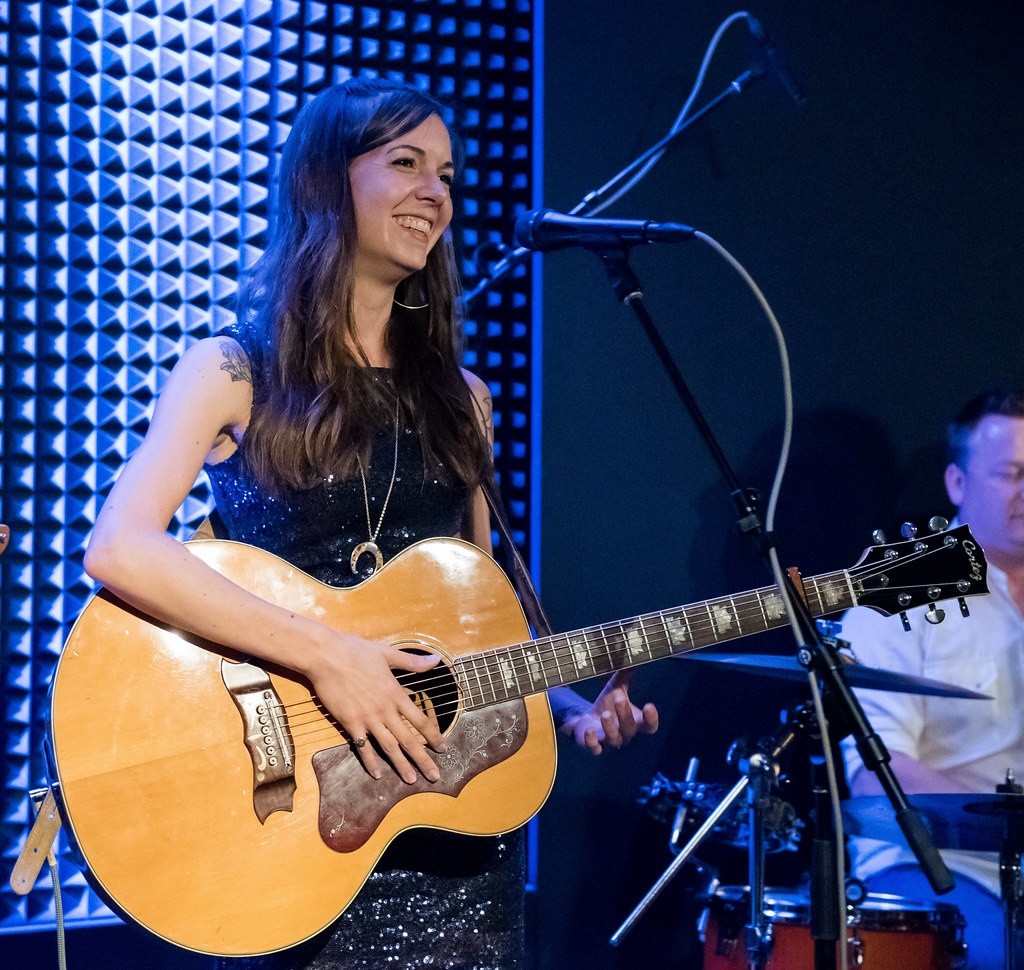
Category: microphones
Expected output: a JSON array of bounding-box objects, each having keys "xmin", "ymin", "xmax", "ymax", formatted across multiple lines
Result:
[
  {"xmin": 515, "ymin": 209, "xmax": 699, "ymax": 252},
  {"xmin": 670, "ymin": 758, "xmax": 699, "ymax": 843},
  {"xmin": 750, "ymin": 18, "xmax": 810, "ymax": 104}
]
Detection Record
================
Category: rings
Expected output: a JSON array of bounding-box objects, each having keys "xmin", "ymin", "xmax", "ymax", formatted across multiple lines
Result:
[{"xmin": 352, "ymin": 737, "xmax": 368, "ymax": 749}]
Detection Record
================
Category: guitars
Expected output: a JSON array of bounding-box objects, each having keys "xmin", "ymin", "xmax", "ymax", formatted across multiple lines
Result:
[{"xmin": 43, "ymin": 514, "xmax": 994, "ymax": 959}]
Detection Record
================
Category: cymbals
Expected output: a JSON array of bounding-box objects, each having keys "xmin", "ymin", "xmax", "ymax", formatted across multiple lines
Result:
[
  {"xmin": 824, "ymin": 789, "xmax": 1024, "ymax": 855},
  {"xmin": 666, "ymin": 634, "xmax": 995, "ymax": 703}
]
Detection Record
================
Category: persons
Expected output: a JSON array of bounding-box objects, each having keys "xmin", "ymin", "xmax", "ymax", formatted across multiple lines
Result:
[
  {"xmin": 835, "ymin": 398, "xmax": 1024, "ymax": 970},
  {"xmin": 83, "ymin": 82, "xmax": 658, "ymax": 970}
]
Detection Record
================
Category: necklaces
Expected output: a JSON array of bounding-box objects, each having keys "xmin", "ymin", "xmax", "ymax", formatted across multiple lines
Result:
[{"xmin": 350, "ymin": 397, "xmax": 400, "ymax": 574}]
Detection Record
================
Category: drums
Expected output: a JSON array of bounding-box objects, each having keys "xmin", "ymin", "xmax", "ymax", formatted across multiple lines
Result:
[{"xmin": 688, "ymin": 883, "xmax": 966, "ymax": 970}]
